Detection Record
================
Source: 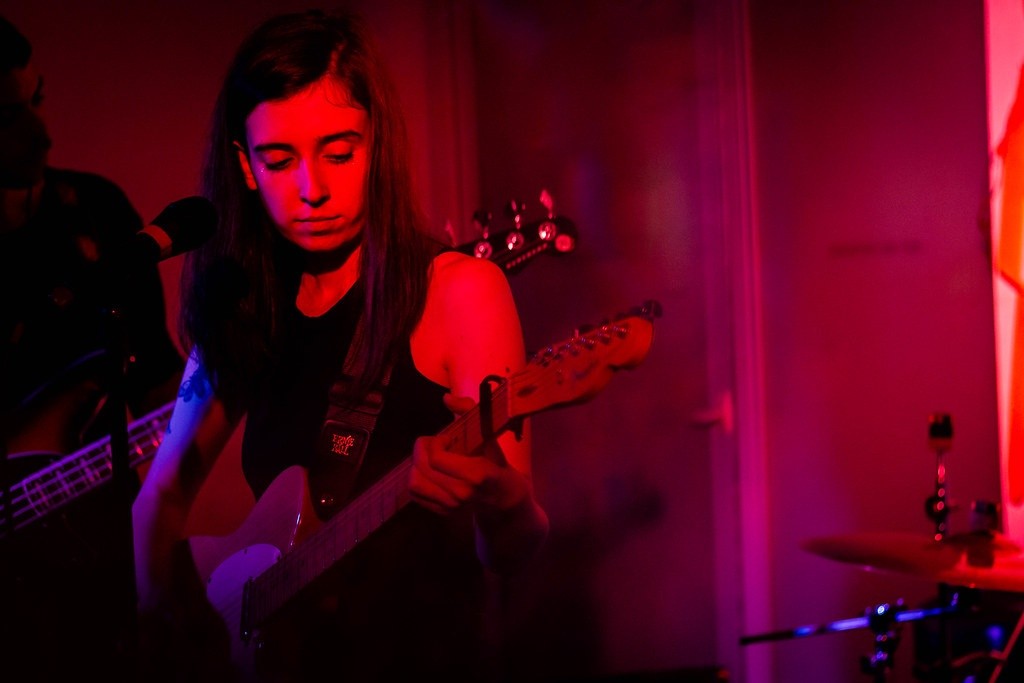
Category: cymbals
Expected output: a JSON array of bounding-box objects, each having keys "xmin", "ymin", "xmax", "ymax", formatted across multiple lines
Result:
[{"xmin": 795, "ymin": 534, "xmax": 1023, "ymax": 605}]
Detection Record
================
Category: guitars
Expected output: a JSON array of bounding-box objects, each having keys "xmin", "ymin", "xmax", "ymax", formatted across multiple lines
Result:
[
  {"xmin": 1, "ymin": 186, "xmax": 579, "ymax": 640},
  {"xmin": 138, "ymin": 297, "xmax": 667, "ymax": 683}
]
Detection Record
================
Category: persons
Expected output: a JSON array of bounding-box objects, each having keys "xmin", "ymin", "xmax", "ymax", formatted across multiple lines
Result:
[
  {"xmin": 0, "ymin": 16, "xmax": 202, "ymax": 682},
  {"xmin": 129, "ymin": 2, "xmax": 556, "ymax": 682}
]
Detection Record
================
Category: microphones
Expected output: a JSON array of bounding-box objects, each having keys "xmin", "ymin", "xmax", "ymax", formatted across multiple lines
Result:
[{"xmin": 47, "ymin": 196, "xmax": 219, "ymax": 315}]
